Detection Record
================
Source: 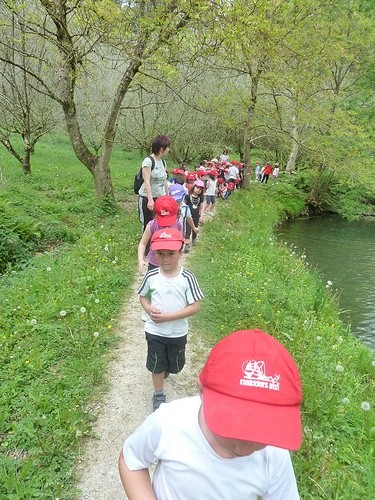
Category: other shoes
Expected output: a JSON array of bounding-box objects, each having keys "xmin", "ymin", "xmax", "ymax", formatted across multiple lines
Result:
[{"xmin": 210, "ymin": 211, "xmax": 214, "ymax": 216}]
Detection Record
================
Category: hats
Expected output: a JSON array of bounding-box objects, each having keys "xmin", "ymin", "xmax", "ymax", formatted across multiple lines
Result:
[
  {"xmin": 214, "ymin": 164, "xmax": 219, "ymax": 167},
  {"xmin": 193, "ymin": 180, "xmax": 204, "ymax": 188},
  {"xmin": 256, "ymin": 162, "xmax": 260, "ymax": 164},
  {"xmin": 169, "ymin": 183, "xmax": 187, "ymax": 202},
  {"xmin": 218, "ymin": 178, "xmax": 223, "ymax": 184},
  {"xmin": 166, "ymin": 167, "xmax": 218, "ymax": 181},
  {"xmin": 222, "ymin": 161, "xmax": 226, "ymax": 165},
  {"xmin": 151, "ymin": 227, "xmax": 184, "ymax": 250},
  {"xmin": 199, "ymin": 328, "xmax": 302, "ymax": 451},
  {"xmin": 228, "ymin": 182, "xmax": 234, "ymax": 191},
  {"xmin": 275, "ymin": 163, "xmax": 279, "ymax": 166},
  {"xmin": 153, "ymin": 196, "xmax": 178, "ymax": 227},
  {"xmin": 230, "ymin": 161, "xmax": 243, "ymax": 168},
  {"xmin": 212, "ymin": 159, "xmax": 218, "ymax": 163}
]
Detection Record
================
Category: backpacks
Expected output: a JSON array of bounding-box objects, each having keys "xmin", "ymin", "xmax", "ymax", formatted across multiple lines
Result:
[{"xmin": 133, "ymin": 155, "xmax": 165, "ymax": 195}]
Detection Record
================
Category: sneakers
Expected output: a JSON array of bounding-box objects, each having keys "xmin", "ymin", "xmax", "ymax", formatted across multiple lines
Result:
[
  {"xmin": 184, "ymin": 243, "xmax": 189, "ymax": 253},
  {"xmin": 141, "ymin": 311, "xmax": 148, "ymax": 322},
  {"xmin": 164, "ymin": 371, "xmax": 170, "ymax": 379},
  {"xmin": 153, "ymin": 393, "xmax": 166, "ymax": 412},
  {"xmin": 192, "ymin": 238, "xmax": 196, "ymax": 246}
]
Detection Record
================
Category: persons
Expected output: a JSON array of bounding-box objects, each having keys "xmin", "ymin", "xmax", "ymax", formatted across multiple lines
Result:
[
  {"xmin": 166, "ymin": 158, "xmax": 254, "ymax": 199},
  {"xmin": 118, "ymin": 328, "xmax": 304, "ymax": 499},
  {"xmin": 219, "ymin": 151, "xmax": 229, "ymax": 162},
  {"xmin": 260, "ymin": 163, "xmax": 267, "ymax": 183},
  {"xmin": 169, "ymin": 183, "xmax": 200, "ymax": 253},
  {"xmin": 202, "ymin": 170, "xmax": 219, "ymax": 215},
  {"xmin": 272, "ymin": 163, "xmax": 279, "ymax": 185},
  {"xmin": 137, "ymin": 226, "xmax": 205, "ymax": 411},
  {"xmin": 138, "ymin": 195, "xmax": 192, "ymax": 322},
  {"xmin": 240, "ymin": 153, "xmax": 245, "ymax": 163},
  {"xmin": 254, "ymin": 162, "xmax": 260, "ymax": 182},
  {"xmin": 137, "ymin": 135, "xmax": 171, "ymax": 257},
  {"xmin": 260, "ymin": 162, "xmax": 272, "ymax": 184},
  {"xmin": 183, "ymin": 180, "xmax": 204, "ymax": 245}
]
